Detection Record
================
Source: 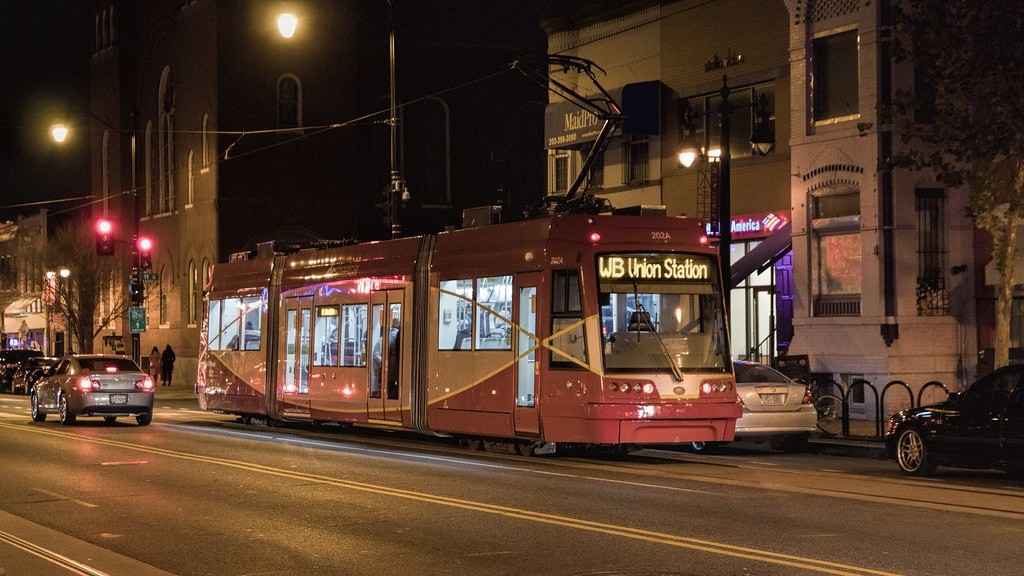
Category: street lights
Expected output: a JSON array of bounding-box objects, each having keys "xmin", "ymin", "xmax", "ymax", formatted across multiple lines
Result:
[
  {"xmin": 679, "ymin": 69, "xmax": 734, "ymax": 351},
  {"xmin": 58, "ymin": 262, "xmax": 77, "ymax": 356},
  {"xmin": 44, "ymin": 268, "xmax": 58, "ymax": 358},
  {"xmin": 50, "ymin": 104, "xmax": 141, "ymax": 369}
]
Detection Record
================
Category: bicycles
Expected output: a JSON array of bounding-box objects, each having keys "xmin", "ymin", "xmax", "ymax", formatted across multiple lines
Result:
[{"xmin": 801, "ymin": 377, "xmax": 847, "ymax": 436}]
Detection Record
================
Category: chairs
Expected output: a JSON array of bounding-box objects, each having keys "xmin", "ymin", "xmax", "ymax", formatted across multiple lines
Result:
[
  {"xmin": 628, "ymin": 311, "xmax": 652, "ymax": 333},
  {"xmin": 226, "ymin": 332, "xmax": 366, "ymax": 392},
  {"xmin": 459, "ymin": 333, "xmax": 511, "ymax": 350}
]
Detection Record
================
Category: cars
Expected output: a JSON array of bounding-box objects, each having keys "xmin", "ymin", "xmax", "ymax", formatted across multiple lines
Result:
[
  {"xmin": 883, "ymin": 358, "xmax": 1024, "ymax": 481},
  {"xmin": 29, "ymin": 353, "xmax": 156, "ymax": 427},
  {"xmin": 642, "ymin": 358, "xmax": 819, "ymax": 454},
  {"xmin": 10, "ymin": 356, "xmax": 58, "ymax": 395}
]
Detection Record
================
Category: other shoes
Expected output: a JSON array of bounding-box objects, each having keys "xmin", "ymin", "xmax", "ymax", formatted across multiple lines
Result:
[
  {"xmin": 168, "ymin": 383, "xmax": 170, "ymax": 386},
  {"xmin": 162, "ymin": 383, "xmax": 166, "ymax": 386}
]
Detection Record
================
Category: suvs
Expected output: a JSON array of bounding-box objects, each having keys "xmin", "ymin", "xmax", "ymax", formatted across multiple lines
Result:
[{"xmin": 0, "ymin": 349, "xmax": 46, "ymax": 394}]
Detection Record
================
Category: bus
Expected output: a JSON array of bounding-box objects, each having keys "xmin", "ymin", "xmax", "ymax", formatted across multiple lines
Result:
[{"xmin": 183, "ymin": 48, "xmax": 745, "ymax": 464}]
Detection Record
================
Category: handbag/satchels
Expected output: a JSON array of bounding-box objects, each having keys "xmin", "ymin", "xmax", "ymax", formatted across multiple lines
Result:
[{"xmin": 149, "ymin": 365, "xmax": 155, "ymax": 369}]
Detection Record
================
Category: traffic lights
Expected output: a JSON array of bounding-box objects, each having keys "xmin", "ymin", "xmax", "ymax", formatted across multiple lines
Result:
[
  {"xmin": 141, "ymin": 238, "xmax": 153, "ymax": 270},
  {"xmin": 96, "ymin": 217, "xmax": 115, "ymax": 256}
]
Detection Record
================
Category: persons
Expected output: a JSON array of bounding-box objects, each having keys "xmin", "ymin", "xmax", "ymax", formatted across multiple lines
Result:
[
  {"xmin": 160, "ymin": 344, "xmax": 176, "ymax": 386},
  {"xmin": 149, "ymin": 346, "xmax": 161, "ymax": 384},
  {"xmin": 374, "ymin": 318, "xmax": 400, "ymax": 400}
]
